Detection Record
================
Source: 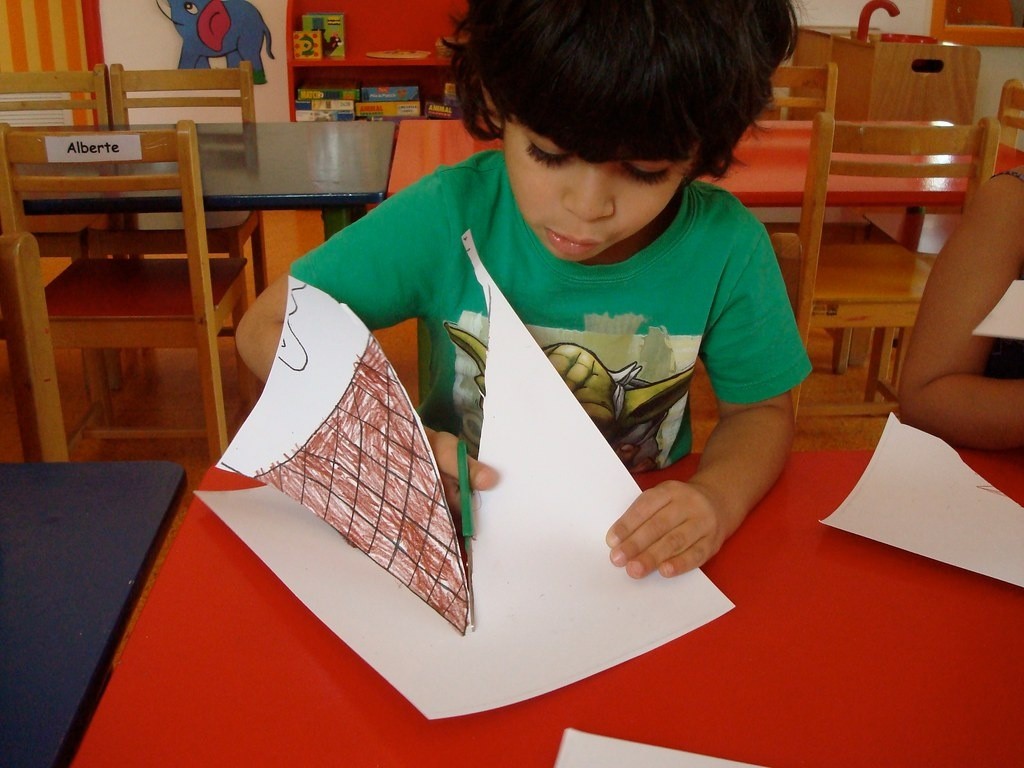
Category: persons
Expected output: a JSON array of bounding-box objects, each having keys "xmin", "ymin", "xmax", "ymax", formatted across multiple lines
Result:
[
  {"xmin": 235, "ymin": 2, "xmax": 814, "ymax": 578},
  {"xmin": 895, "ymin": 156, "xmax": 1024, "ymax": 452}
]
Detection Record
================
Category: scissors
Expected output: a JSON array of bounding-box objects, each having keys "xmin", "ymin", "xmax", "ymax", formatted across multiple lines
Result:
[{"xmin": 455, "ymin": 437, "xmax": 476, "ymax": 631}]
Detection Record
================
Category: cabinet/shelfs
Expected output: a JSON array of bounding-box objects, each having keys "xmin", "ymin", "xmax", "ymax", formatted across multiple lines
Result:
[{"xmin": 786, "ymin": 25, "xmax": 980, "ymax": 223}]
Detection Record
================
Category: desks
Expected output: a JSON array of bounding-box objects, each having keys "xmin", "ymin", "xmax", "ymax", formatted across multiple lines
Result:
[
  {"xmin": 0, "ymin": 121, "xmax": 396, "ymax": 241},
  {"xmin": 71, "ymin": 450, "xmax": 1024, "ymax": 768},
  {"xmin": 386, "ymin": 120, "xmax": 1024, "ymax": 397},
  {"xmin": 0, "ymin": 462, "xmax": 187, "ymax": 768}
]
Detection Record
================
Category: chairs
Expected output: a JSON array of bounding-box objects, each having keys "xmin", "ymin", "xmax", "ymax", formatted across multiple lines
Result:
[
  {"xmin": 0, "ymin": 63, "xmax": 126, "ymax": 261},
  {"xmin": 109, "ymin": 62, "xmax": 268, "ymax": 390},
  {"xmin": 0, "ymin": 231, "xmax": 69, "ymax": 463},
  {"xmin": 996, "ymin": 78, "xmax": 1024, "ymax": 149},
  {"xmin": 0, "ymin": 121, "xmax": 250, "ymax": 464},
  {"xmin": 755, "ymin": 62, "xmax": 839, "ymax": 123},
  {"xmin": 773, "ymin": 111, "xmax": 998, "ymax": 417}
]
